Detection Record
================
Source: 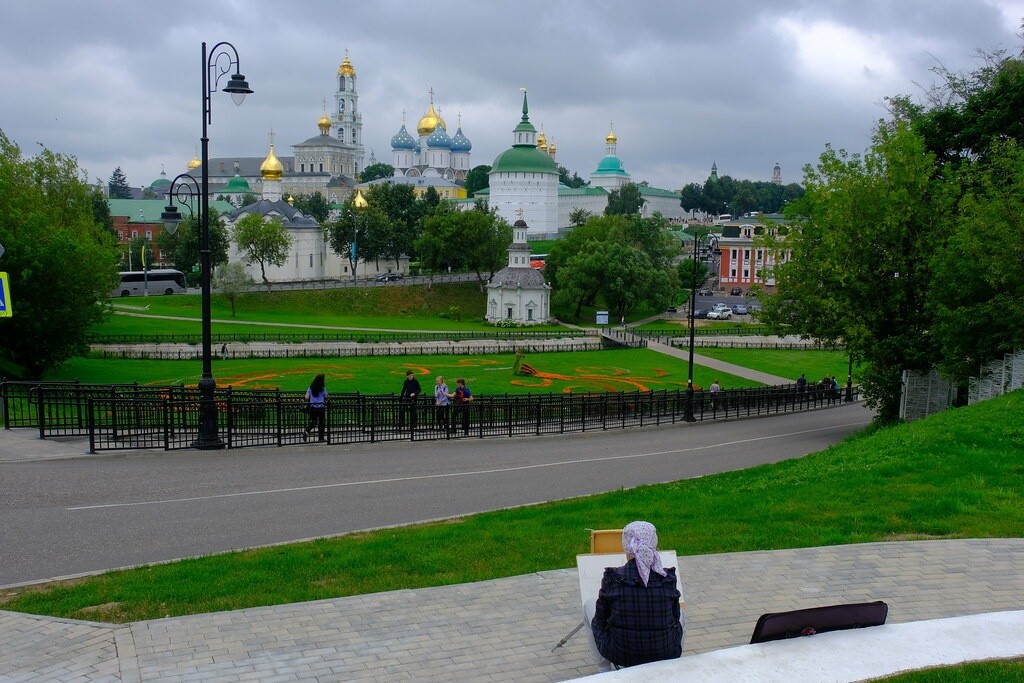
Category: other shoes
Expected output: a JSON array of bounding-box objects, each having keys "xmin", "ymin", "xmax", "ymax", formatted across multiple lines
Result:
[
  {"xmin": 319, "ymin": 438, "xmax": 327, "ymax": 441},
  {"xmin": 719, "ymin": 407, "xmax": 722, "ymax": 409},
  {"xmin": 303, "ymin": 431, "xmax": 307, "ymax": 441}
]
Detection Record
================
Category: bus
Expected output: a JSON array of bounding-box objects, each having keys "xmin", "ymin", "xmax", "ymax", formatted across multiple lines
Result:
[
  {"xmin": 101, "ymin": 268, "xmax": 188, "ymax": 297},
  {"xmin": 530, "ymin": 254, "xmax": 551, "ymax": 270}
]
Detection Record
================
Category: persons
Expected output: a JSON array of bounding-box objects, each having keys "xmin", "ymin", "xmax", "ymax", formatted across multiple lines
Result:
[
  {"xmin": 583, "ymin": 521, "xmax": 687, "ymax": 673},
  {"xmin": 434, "ymin": 376, "xmax": 450, "ymax": 429},
  {"xmin": 221, "ymin": 343, "xmax": 228, "ymax": 361},
  {"xmin": 302, "ymin": 373, "xmax": 328, "ymax": 441},
  {"xmin": 710, "ymin": 379, "xmax": 720, "ymax": 412},
  {"xmin": 439, "ymin": 378, "xmax": 475, "ymax": 435},
  {"xmin": 797, "ymin": 373, "xmax": 838, "ymax": 400},
  {"xmin": 397, "ymin": 370, "xmax": 421, "ymax": 432}
]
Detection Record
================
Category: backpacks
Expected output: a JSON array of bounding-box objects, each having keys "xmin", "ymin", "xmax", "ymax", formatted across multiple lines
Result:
[{"xmin": 454, "ymin": 387, "xmax": 469, "ymax": 405}]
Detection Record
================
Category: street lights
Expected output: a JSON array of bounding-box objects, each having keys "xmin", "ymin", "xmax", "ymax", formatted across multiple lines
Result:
[
  {"xmin": 160, "ymin": 39, "xmax": 254, "ymax": 453},
  {"xmin": 667, "ymin": 231, "xmax": 723, "ymax": 422}
]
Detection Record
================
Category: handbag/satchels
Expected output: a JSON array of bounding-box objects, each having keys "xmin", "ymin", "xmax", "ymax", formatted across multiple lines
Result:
[{"xmin": 300, "ymin": 397, "xmax": 310, "ymax": 413}]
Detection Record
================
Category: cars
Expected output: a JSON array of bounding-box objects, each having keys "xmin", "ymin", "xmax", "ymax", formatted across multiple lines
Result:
[
  {"xmin": 694, "ymin": 303, "xmax": 761, "ymax": 320},
  {"xmin": 730, "ymin": 286, "xmax": 743, "ymax": 296},
  {"xmin": 699, "ymin": 288, "xmax": 713, "ymax": 297},
  {"xmin": 376, "ymin": 272, "xmax": 401, "ymax": 282},
  {"xmin": 745, "ymin": 289, "xmax": 758, "ymax": 296}
]
